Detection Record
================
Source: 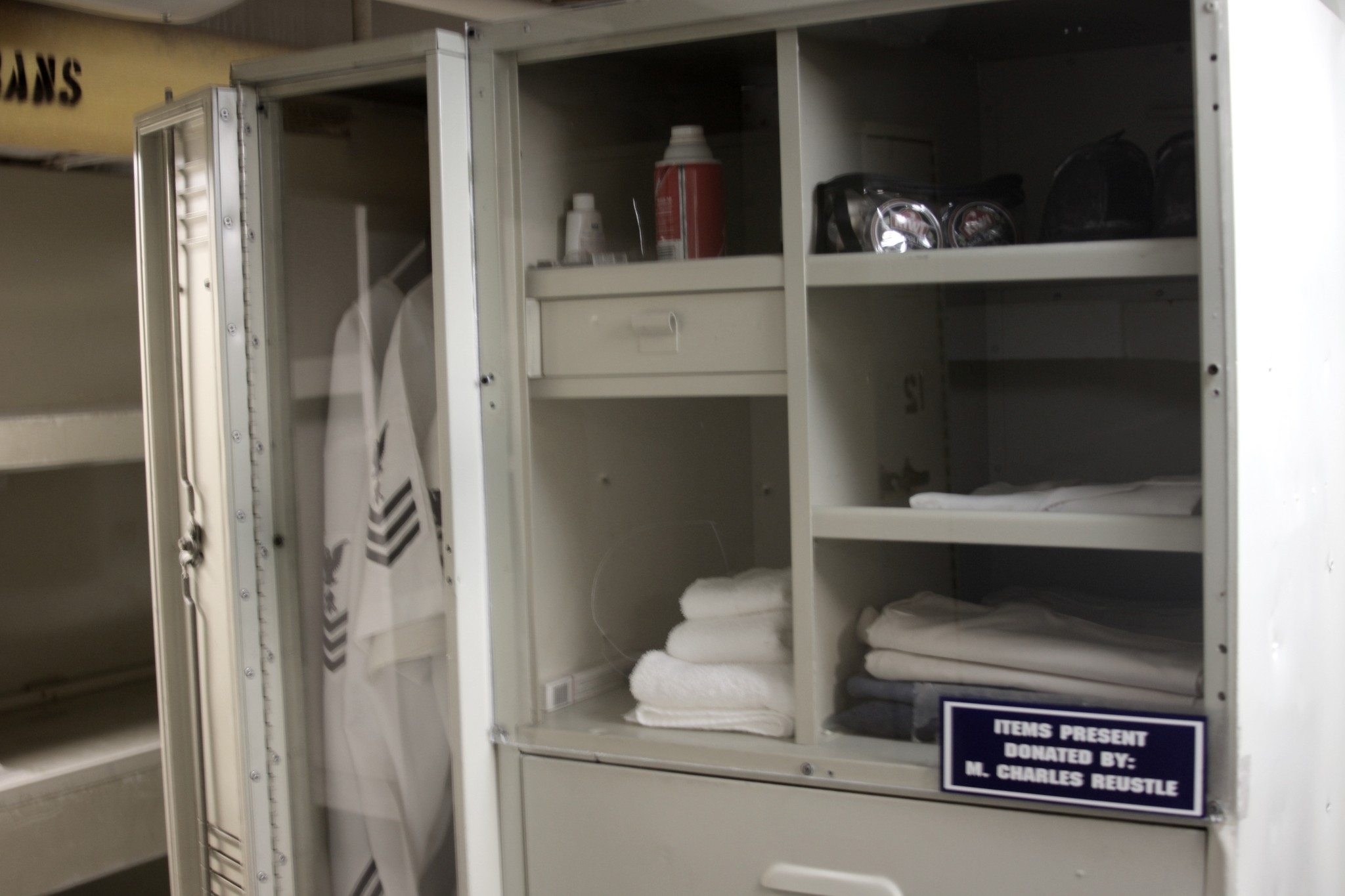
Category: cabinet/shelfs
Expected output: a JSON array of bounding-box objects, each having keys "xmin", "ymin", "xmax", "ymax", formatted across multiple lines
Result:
[{"xmin": 466, "ymin": 2, "xmax": 1236, "ymax": 896}]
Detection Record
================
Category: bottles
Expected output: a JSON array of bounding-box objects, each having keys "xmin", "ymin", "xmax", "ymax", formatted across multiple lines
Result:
[
  {"xmin": 566, "ymin": 193, "xmax": 608, "ymax": 267},
  {"xmin": 657, "ymin": 127, "xmax": 726, "ymax": 261}
]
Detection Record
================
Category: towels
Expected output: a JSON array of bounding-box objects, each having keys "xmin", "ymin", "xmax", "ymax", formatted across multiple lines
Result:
[{"xmin": 622, "ymin": 565, "xmax": 796, "ymax": 743}]
